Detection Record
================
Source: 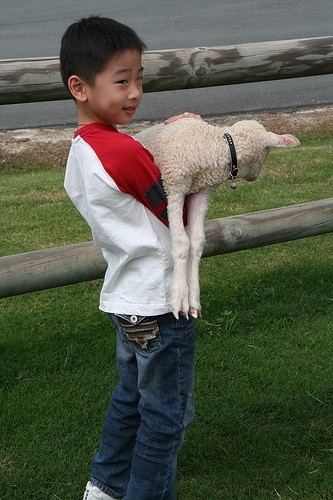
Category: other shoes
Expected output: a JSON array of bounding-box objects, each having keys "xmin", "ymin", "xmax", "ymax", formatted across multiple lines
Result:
[{"xmin": 82, "ymin": 481, "xmax": 118, "ymax": 500}]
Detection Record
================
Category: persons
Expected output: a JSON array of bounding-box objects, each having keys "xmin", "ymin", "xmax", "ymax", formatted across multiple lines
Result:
[{"xmin": 62, "ymin": 11, "xmax": 203, "ymax": 500}]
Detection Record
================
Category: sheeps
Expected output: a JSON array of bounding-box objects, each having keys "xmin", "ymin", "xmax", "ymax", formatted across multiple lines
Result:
[{"xmin": 129, "ymin": 117, "xmax": 301, "ymax": 322}]
